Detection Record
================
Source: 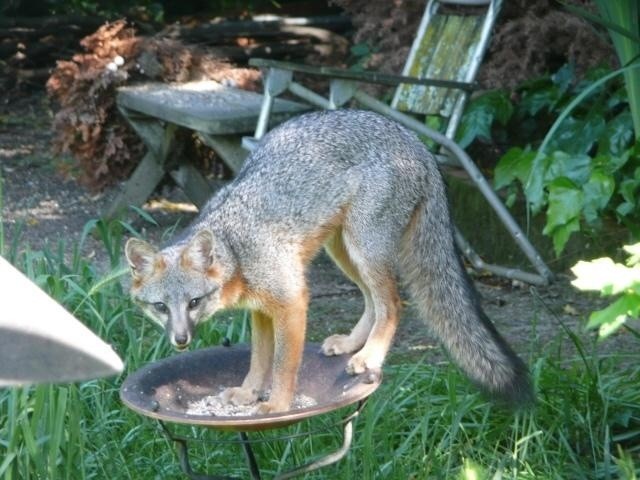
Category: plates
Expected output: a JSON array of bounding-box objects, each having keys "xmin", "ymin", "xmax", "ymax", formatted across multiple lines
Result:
[{"xmin": 117, "ymin": 341, "xmax": 385, "ymax": 433}]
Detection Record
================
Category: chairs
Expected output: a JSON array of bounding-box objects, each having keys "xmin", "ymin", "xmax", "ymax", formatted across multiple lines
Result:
[{"xmin": 241, "ymin": 0, "xmax": 554, "ymax": 287}]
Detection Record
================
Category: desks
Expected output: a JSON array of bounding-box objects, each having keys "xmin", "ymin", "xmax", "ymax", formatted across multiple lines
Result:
[{"xmin": 105, "ymin": 81, "xmax": 313, "ymax": 230}]
